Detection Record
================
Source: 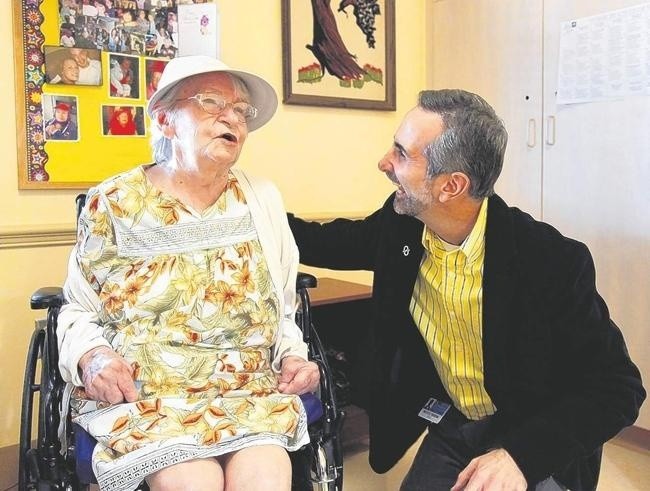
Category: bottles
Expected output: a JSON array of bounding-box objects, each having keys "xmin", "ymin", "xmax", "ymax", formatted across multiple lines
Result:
[
  {"xmin": 53, "ymin": 103, "xmax": 72, "ymax": 112},
  {"xmin": 146, "ymin": 54, "xmax": 279, "ymax": 133}
]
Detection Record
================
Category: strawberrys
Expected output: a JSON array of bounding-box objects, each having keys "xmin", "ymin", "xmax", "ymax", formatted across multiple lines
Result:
[{"xmin": 17, "ymin": 270, "xmax": 343, "ymax": 491}]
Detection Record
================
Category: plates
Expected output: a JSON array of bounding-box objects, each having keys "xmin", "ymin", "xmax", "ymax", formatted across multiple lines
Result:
[{"xmin": 296, "ymin": 279, "xmax": 382, "ymax": 459}]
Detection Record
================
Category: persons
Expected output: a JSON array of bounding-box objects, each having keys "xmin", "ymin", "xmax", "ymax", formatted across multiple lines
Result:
[
  {"xmin": 286, "ymin": 90, "xmax": 647, "ymax": 491},
  {"xmin": 57, "ymin": 55, "xmax": 321, "ymax": 491},
  {"xmin": 45, "ymin": 0, "xmax": 179, "ymax": 139}
]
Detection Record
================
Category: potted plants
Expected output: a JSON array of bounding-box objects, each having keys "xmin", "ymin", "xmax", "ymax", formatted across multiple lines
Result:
[
  {"xmin": 10, "ymin": 0, "xmax": 216, "ymax": 193},
  {"xmin": 279, "ymin": 1, "xmax": 397, "ymax": 112}
]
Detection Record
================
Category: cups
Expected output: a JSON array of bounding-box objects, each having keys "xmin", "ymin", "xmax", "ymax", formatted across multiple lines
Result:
[{"xmin": 163, "ymin": 91, "xmax": 258, "ymax": 125}]
